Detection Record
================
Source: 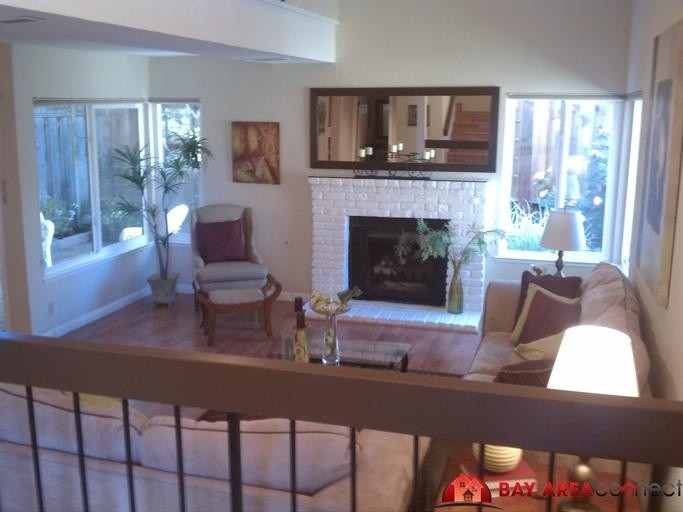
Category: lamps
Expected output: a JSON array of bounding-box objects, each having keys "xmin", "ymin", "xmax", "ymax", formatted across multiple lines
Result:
[
  {"xmin": 538, "ymin": 207, "xmax": 582, "ymax": 277},
  {"xmin": 536, "ymin": 322, "xmax": 643, "ymax": 512}
]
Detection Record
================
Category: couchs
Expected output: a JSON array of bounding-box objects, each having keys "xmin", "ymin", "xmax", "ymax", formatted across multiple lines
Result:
[
  {"xmin": 1, "ymin": 378, "xmax": 432, "ymax": 511},
  {"xmin": 428, "ymin": 261, "xmax": 660, "ymax": 511}
]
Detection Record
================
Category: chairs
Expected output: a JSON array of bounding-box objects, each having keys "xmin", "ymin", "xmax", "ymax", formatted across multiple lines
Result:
[{"xmin": 186, "ymin": 203, "xmax": 282, "ymax": 346}]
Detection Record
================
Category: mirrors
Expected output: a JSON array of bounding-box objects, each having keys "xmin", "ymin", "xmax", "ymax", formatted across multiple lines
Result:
[{"xmin": 308, "ymin": 84, "xmax": 500, "ymax": 174}]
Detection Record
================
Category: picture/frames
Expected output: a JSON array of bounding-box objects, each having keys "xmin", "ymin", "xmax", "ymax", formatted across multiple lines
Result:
[{"xmin": 229, "ymin": 120, "xmax": 282, "ymax": 186}]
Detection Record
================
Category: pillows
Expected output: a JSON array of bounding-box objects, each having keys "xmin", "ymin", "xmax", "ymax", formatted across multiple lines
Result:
[
  {"xmin": 192, "ymin": 218, "xmax": 246, "ymax": 265},
  {"xmin": 492, "ymin": 269, "xmax": 584, "ymax": 396}
]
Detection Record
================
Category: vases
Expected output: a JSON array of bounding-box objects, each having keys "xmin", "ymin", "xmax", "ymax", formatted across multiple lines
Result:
[{"xmin": 320, "ymin": 313, "xmax": 340, "ymax": 365}]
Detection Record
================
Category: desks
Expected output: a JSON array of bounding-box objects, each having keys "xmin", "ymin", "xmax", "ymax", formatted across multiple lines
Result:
[{"xmin": 432, "ymin": 455, "xmax": 642, "ymax": 512}]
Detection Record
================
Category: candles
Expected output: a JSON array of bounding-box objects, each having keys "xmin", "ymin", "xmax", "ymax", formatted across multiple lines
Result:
[
  {"xmin": 421, "ymin": 151, "xmax": 430, "ymax": 161},
  {"xmin": 395, "ymin": 142, "xmax": 404, "ymax": 152},
  {"xmin": 427, "ymin": 150, "xmax": 435, "ymax": 159},
  {"xmin": 388, "ymin": 144, "xmax": 397, "ymax": 154},
  {"xmin": 355, "ymin": 148, "xmax": 366, "ymax": 158},
  {"xmin": 364, "ymin": 147, "xmax": 373, "ymax": 156}
]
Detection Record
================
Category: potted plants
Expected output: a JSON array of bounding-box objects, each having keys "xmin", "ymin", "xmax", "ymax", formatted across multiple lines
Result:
[
  {"xmin": 413, "ymin": 212, "xmax": 512, "ymax": 316},
  {"xmin": 106, "ymin": 124, "xmax": 217, "ymax": 306}
]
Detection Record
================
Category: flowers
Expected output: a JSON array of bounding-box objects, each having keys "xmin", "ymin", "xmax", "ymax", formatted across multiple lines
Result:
[{"xmin": 308, "ymin": 285, "xmax": 364, "ymax": 360}]
